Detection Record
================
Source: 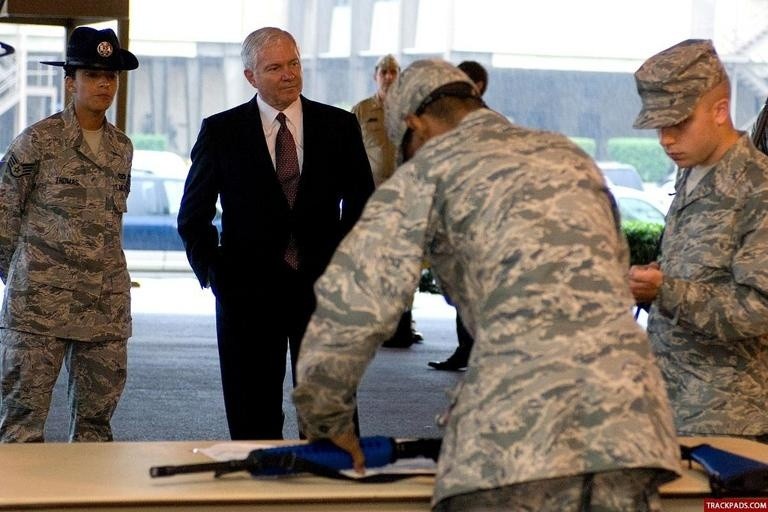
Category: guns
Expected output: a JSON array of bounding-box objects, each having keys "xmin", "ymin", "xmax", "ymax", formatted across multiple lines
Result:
[{"xmin": 149, "ymin": 437, "xmax": 768, "ymax": 496}]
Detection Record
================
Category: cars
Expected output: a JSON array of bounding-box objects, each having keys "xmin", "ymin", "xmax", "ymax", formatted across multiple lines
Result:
[
  {"xmin": 596, "ymin": 162, "xmax": 666, "ymax": 227},
  {"xmin": 121, "ymin": 150, "xmax": 222, "ymax": 249}
]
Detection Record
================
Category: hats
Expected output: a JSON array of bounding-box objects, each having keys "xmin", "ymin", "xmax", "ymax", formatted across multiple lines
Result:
[
  {"xmin": 384, "ymin": 58, "xmax": 480, "ymax": 163},
  {"xmin": 632, "ymin": 38, "xmax": 729, "ymax": 129},
  {"xmin": 38, "ymin": 26, "xmax": 140, "ymax": 71},
  {"xmin": 374, "ymin": 53, "xmax": 400, "ymax": 70}
]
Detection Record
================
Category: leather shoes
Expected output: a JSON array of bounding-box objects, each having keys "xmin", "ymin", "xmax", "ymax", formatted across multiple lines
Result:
[{"xmin": 427, "ymin": 347, "xmax": 469, "ymax": 369}]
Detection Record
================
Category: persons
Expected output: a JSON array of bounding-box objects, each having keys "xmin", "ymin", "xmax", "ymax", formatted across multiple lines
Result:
[
  {"xmin": 1, "ymin": 25, "xmax": 135, "ymax": 443},
  {"xmin": 349, "ymin": 51, "xmax": 423, "ymax": 349},
  {"xmin": 428, "ymin": 56, "xmax": 489, "ymax": 368},
  {"xmin": 285, "ymin": 52, "xmax": 681, "ymax": 511},
  {"xmin": 624, "ymin": 36, "xmax": 766, "ymax": 444},
  {"xmin": 177, "ymin": 24, "xmax": 374, "ymax": 441}
]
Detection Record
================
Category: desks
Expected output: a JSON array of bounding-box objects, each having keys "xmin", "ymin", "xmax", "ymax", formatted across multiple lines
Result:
[{"xmin": 0, "ymin": 435, "xmax": 768, "ymax": 510}]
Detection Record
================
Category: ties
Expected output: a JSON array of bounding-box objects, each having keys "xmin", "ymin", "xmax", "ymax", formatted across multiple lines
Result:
[{"xmin": 275, "ymin": 113, "xmax": 301, "ymax": 273}]
